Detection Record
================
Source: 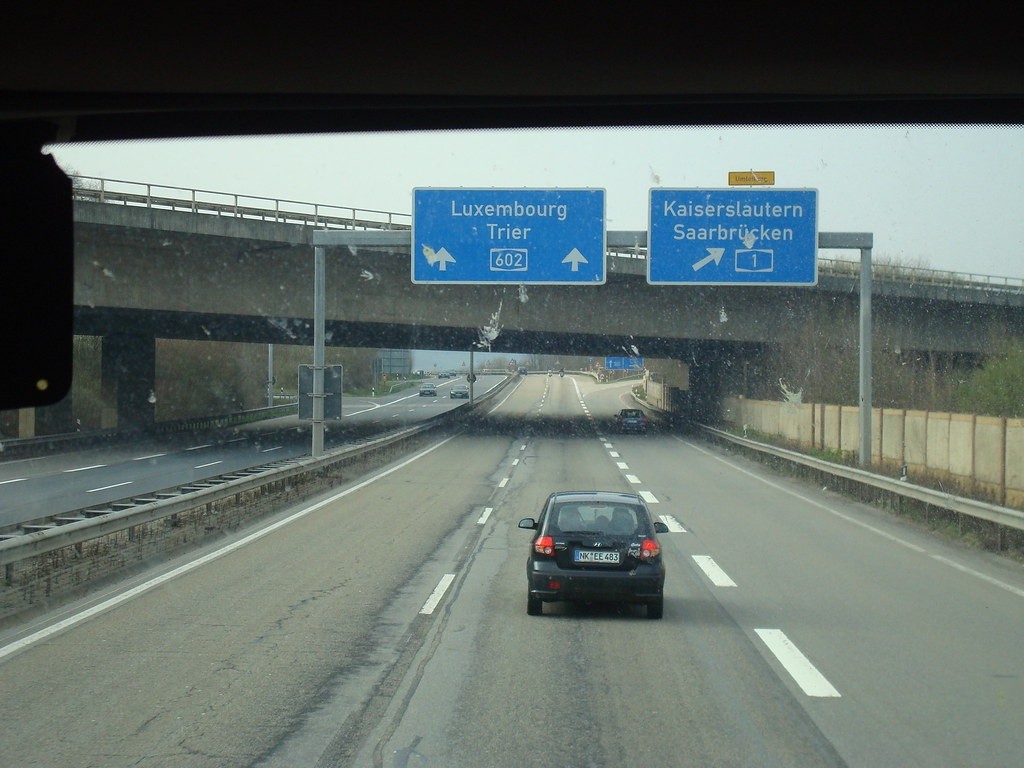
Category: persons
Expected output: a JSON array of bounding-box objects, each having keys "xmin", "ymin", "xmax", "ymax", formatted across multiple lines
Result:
[
  {"xmin": 548, "ymin": 368, "xmax": 551, "ymax": 372},
  {"xmin": 559, "ymin": 369, "xmax": 564, "ymax": 372},
  {"xmin": 597, "ymin": 515, "xmax": 609, "ymax": 529},
  {"xmin": 612, "ymin": 508, "xmax": 629, "ymax": 518}
]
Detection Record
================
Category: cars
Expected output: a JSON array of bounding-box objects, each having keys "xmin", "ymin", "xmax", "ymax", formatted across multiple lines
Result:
[
  {"xmin": 614, "ymin": 408, "xmax": 648, "ymax": 435},
  {"xmin": 517, "ymin": 491, "xmax": 670, "ymax": 620},
  {"xmin": 438, "ymin": 370, "xmax": 450, "ymax": 379},
  {"xmin": 450, "ymin": 384, "xmax": 470, "ymax": 399},
  {"xmin": 420, "ymin": 383, "xmax": 436, "ymax": 396},
  {"xmin": 448, "ymin": 370, "xmax": 456, "ymax": 377},
  {"xmin": 517, "ymin": 366, "xmax": 527, "ymax": 375}
]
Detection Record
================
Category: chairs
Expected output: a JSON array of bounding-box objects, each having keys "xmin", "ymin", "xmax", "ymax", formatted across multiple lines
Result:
[
  {"xmin": 606, "ymin": 507, "xmax": 634, "ymax": 535},
  {"xmin": 623, "ymin": 412, "xmax": 628, "ymax": 418},
  {"xmin": 635, "ymin": 412, "xmax": 639, "ymax": 417},
  {"xmin": 560, "ymin": 507, "xmax": 584, "ymax": 532}
]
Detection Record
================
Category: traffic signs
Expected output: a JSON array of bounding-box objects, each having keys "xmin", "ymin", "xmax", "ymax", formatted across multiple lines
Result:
[
  {"xmin": 410, "ymin": 186, "xmax": 606, "ymax": 285},
  {"xmin": 647, "ymin": 187, "xmax": 820, "ymax": 287}
]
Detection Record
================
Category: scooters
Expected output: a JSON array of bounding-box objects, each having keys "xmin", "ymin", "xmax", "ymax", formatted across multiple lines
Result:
[
  {"xmin": 548, "ymin": 372, "xmax": 552, "ymax": 377},
  {"xmin": 558, "ymin": 371, "xmax": 564, "ymax": 377}
]
